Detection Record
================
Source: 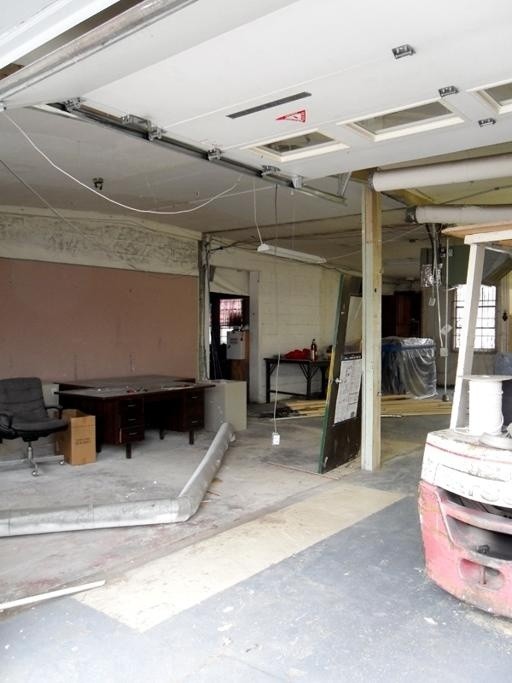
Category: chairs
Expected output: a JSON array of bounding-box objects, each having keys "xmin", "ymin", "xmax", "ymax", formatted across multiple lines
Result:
[{"xmin": 0, "ymin": 376, "xmax": 70, "ymax": 478}]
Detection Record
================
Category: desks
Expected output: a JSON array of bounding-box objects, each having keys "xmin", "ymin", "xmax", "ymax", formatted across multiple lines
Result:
[
  {"xmin": 52, "ymin": 374, "xmax": 217, "ymax": 460},
  {"xmin": 263, "ymin": 353, "xmax": 332, "ymax": 404}
]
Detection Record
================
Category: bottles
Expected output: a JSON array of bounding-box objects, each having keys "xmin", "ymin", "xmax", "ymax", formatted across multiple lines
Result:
[{"xmin": 310, "ymin": 339, "xmax": 318, "ymax": 361}]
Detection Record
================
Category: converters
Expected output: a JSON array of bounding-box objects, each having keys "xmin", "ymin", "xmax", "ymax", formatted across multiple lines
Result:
[{"xmin": 272, "ymin": 433, "xmax": 281, "ymax": 446}]
